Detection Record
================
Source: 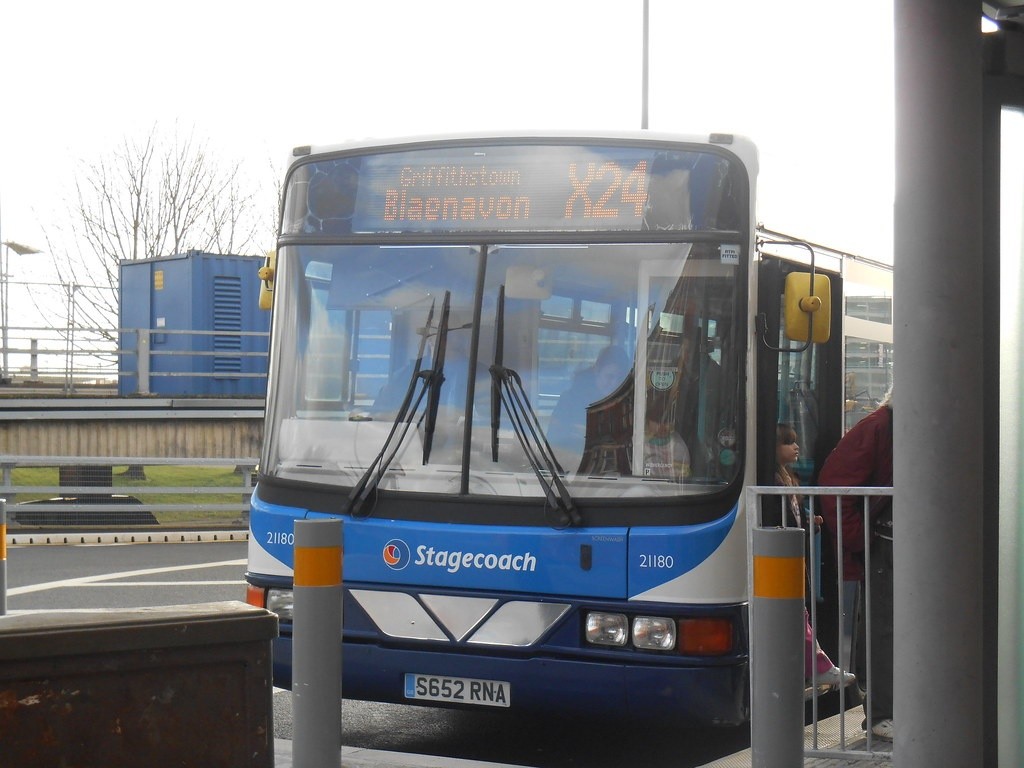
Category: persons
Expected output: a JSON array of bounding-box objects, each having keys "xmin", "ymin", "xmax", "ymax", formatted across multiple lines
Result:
[
  {"xmin": 816, "ymin": 386, "xmax": 893, "ymax": 744},
  {"xmin": 774, "ymin": 424, "xmax": 855, "ymax": 687},
  {"xmin": 369, "ymin": 312, "xmax": 490, "ymax": 419},
  {"xmin": 548, "ymin": 349, "xmax": 632, "ymax": 470},
  {"xmin": 646, "ymin": 397, "xmax": 692, "ymax": 477},
  {"xmin": 669, "ymin": 328, "xmax": 720, "ymax": 439}
]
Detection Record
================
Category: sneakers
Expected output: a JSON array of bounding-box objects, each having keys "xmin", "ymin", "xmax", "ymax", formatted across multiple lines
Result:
[
  {"xmin": 812, "ymin": 667, "xmax": 856, "ymax": 693},
  {"xmin": 871, "ymin": 719, "xmax": 893, "ymax": 741}
]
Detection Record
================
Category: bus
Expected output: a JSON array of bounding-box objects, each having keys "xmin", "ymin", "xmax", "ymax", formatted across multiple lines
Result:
[{"xmin": 240, "ymin": 129, "xmax": 893, "ymax": 748}]
